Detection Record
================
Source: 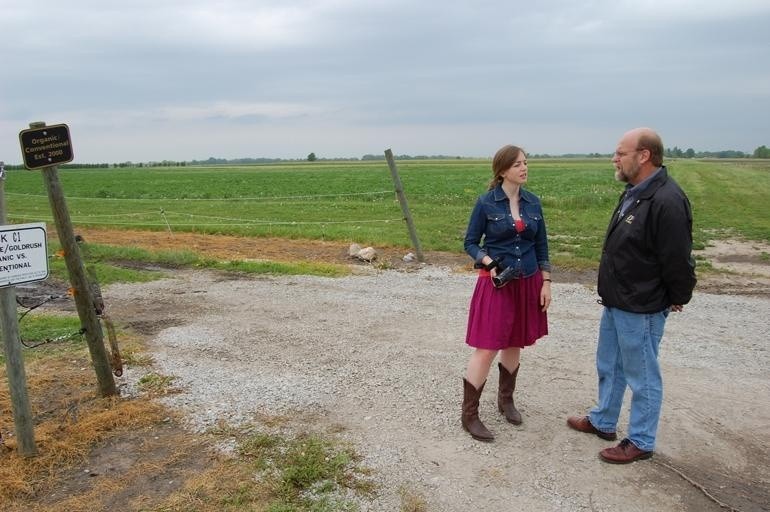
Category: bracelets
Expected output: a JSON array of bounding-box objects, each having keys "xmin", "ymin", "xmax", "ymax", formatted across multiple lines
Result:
[
  {"xmin": 543, "ymin": 279, "xmax": 551, "ymax": 281},
  {"xmin": 487, "ymin": 260, "xmax": 498, "ymax": 271}
]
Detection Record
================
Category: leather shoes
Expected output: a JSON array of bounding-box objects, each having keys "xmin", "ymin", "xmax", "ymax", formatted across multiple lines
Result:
[
  {"xmin": 597, "ymin": 439, "xmax": 654, "ymax": 464},
  {"xmin": 567, "ymin": 415, "xmax": 617, "ymax": 441}
]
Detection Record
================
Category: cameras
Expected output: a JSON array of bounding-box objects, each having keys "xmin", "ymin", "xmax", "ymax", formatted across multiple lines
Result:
[{"xmin": 492, "ymin": 256, "xmax": 515, "ymax": 286}]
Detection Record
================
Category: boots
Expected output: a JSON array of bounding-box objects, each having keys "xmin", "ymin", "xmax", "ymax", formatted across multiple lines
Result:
[
  {"xmin": 497, "ymin": 362, "xmax": 522, "ymax": 424},
  {"xmin": 462, "ymin": 377, "xmax": 495, "ymax": 441}
]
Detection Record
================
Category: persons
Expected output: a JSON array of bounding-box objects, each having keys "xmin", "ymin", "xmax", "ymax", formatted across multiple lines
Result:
[
  {"xmin": 461, "ymin": 145, "xmax": 552, "ymax": 442},
  {"xmin": 567, "ymin": 127, "xmax": 697, "ymax": 464}
]
